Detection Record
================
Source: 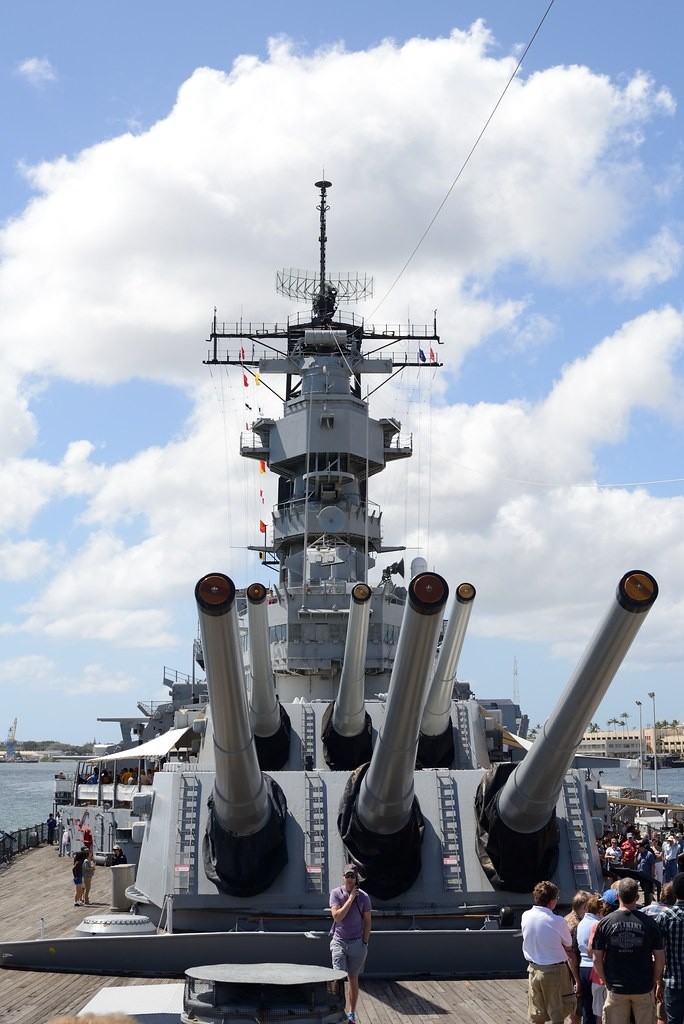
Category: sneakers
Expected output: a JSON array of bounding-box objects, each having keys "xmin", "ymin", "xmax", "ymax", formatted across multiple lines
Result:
[{"xmin": 347, "ymin": 1013, "xmax": 356, "ymax": 1024}]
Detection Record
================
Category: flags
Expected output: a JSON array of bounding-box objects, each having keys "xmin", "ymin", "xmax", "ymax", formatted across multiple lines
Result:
[
  {"xmin": 430, "ymin": 347, "xmax": 435, "ymax": 361},
  {"xmin": 260, "ymin": 460, "xmax": 266, "ymax": 474},
  {"xmin": 258, "ymin": 519, "xmax": 266, "ymax": 560},
  {"xmin": 419, "ymin": 349, "xmax": 426, "ymax": 363},
  {"xmin": 242, "ymin": 344, "xmax": 264, "ymax": 430},
  {"xmin": 260, "ymin": 489, "xmax": 265, "ymax": 504}
]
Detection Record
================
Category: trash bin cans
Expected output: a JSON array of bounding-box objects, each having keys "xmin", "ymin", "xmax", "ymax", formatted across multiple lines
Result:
[{"xmin": 111, "ymin": 864, "xmax": 136, "ymax": 912}]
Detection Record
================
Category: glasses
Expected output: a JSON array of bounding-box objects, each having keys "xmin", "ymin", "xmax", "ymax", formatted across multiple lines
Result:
[
  {"xmin": 343, "ymin": 873, "xmax": 355, "ymax": 880},
  {"xmin": 553, "ymin": 896, "xmax": 560, "ymax": 900},
  {"xmin": 112, "ymin": 849, "xmax": 117, "ymax": 851}
]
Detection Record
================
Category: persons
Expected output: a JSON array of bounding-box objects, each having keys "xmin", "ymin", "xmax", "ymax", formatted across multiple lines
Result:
[
  {"xmin": 72, "ymin": 847, "xmax": 97, "ymax": 907},
  {"xmin": 595, "ymin": 821, "xmax": 684, "ymax": 906},
  {"xmin": 521, "ymin": 881, "xmax": 577, "ymax": 1024},
  {"xmin": 112, "ymin": 845, "xmax": 127, "ymax": 866},
  {"xmin": 266, "ymin": 588, "xmax": 277, "ymax": 605},
  {"xmin": 564, "ymin": 877, "xmax": 666, "ymax": 1024},
  {"xmin": 639, "ymin": 873, "xmax": 684, "ymax": 1024},
  {"xmin": 306, "ymin": 583, "xmax": 311, "ymax": 594},
  {"xmin": 330, "ymin": 864, "xmax": 371, "ymax": 1024},
  {"xmin": 87, "ymin": 767, "xmax": 158, "ymax": 809},
  {"xmin": 47, "ymin": 812, "xmax": 62, "ymax": 845},
  {"xmin": 58, "ymin": 771, "xmax": 87, "ymax": 807}
]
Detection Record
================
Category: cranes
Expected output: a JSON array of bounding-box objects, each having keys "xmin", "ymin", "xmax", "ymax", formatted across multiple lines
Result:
[{"xmin": 5, "ymin": 718, "xmax": 18, "ymax": 759}]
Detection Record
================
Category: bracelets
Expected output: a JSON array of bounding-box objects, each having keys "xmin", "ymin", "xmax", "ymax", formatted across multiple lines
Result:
[{"xmin": 364, "ymin": 942, "xmax": 368, "ymax": 945}]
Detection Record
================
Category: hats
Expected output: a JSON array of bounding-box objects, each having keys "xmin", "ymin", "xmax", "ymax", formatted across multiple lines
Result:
[
  {"xmin": 666, "ymin": 836, "xmax": 674, "ymax": 841},
  {"xmin": 611, "ymin": 838, "xmax": 617, "ymax": 844},
  {"xmin": 597, "ymin": 889, "xmax": 619, "ymax": 906},
  {"xmin": 343, "ymin": 864, "xmax": 358, "ymax": 875},
  {"xmin": 626, "ymin": 832, "xmax": 633, "ymax": 838}
]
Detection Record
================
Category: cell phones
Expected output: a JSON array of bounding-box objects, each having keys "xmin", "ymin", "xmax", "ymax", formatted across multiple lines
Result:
[{"xmin": 356, "ymin": 877, "xmax": 359, "ymax": 890}]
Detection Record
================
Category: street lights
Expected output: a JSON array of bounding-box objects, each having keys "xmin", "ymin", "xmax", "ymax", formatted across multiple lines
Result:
[
  {"xmin": 634, "ymin": 700, "xmax": 644, "ymax": 790},
  {"xmin": 647, "ymin": 692, "xmax": 658, "ymax": 804}
]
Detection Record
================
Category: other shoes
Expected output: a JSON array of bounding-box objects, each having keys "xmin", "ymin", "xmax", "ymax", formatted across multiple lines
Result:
[{"xmin": 73, "ymin": 900, "xmax": 94, "ymax": 908}]
